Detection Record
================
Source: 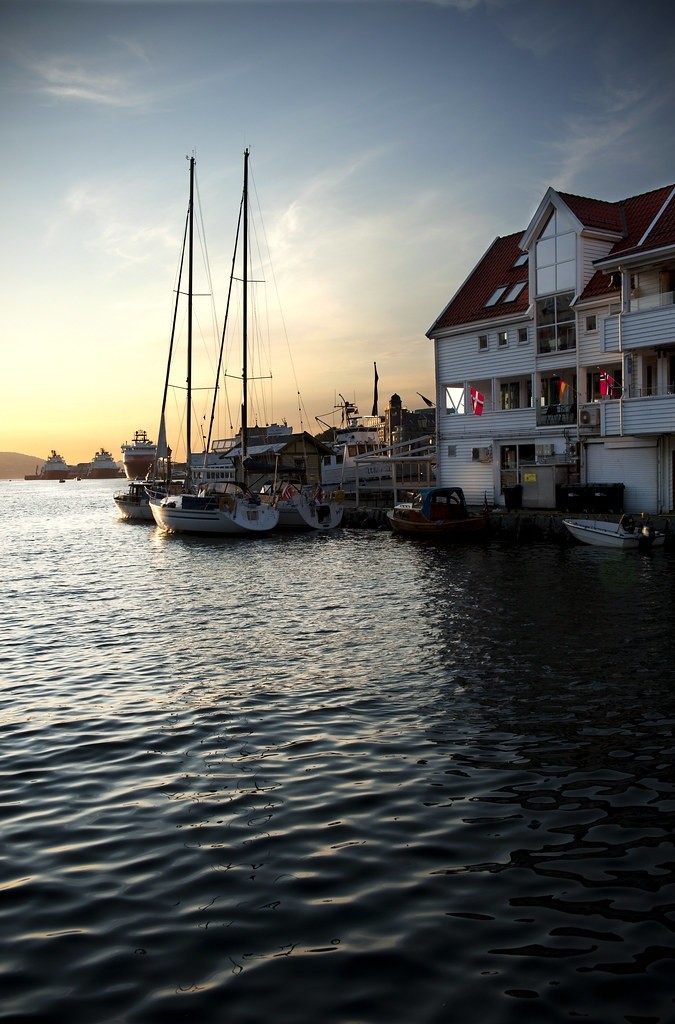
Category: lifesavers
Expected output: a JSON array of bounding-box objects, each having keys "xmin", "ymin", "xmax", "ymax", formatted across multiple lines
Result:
[{"xmin": 219, "ymin": 496, "xmax": 234, "ymax": 512}]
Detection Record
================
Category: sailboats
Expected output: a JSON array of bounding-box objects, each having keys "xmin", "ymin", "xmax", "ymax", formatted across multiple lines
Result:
[
  {"xmin": 109, "ymin": 154, "xmax": 199, "ymax": 522},
  {"xmin": 142, "ymin": 145, "xmax": 282, "ymax": 538}
]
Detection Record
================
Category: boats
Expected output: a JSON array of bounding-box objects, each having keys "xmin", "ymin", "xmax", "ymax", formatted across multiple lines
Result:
[
  {"xmin": 252, "ymin": 473, "xmax": 348, "ymax": 532},
  {"xmin": 117, "ymin": 430, "xmax": 173, "ymax": 481},
  {"xmin": 23, "ymin": 449, "xmax": 71, "ymax": 480},
  {"xmin": 386, "ymin": 486, "xmax": 485, "ymax": 540},
  {"xmin": 561, "ymin": 512, "xmax": 675, "ymax": 550},
  {"xmin": 67, "ymin": 448, "xmax": 121, "ymax": 480}
]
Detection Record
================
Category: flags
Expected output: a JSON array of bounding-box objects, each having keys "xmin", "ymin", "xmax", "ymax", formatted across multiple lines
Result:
[
  {"xmin": 599, "ymin": 371, "xmax": 615, "ymax": 396},
  {"xmin": 557, "ymin": 380, "xmax": 568, "ymax": 401},
  {"xmin": 470, "ymin": 389, "xmax": 486, "ymax": 416}
]
